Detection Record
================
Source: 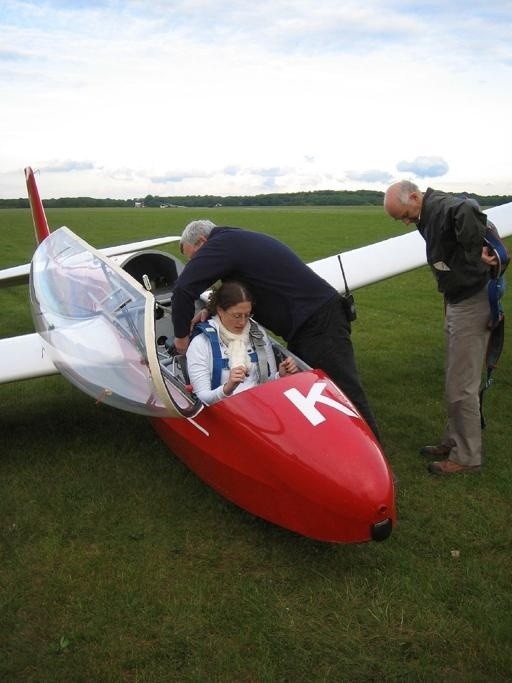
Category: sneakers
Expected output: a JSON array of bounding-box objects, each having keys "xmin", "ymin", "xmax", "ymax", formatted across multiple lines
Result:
[
  {"xmin": 428, "ymin": 459, "xmax": 481, "ymax": 476},
  {"xmin": 420, "ymin": 444, "xmax": 451, "ymax": 458}
]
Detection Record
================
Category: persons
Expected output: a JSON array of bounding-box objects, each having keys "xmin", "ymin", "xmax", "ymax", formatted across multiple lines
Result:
[
  {"xmin": 185, "ymin": 282, "xmax": 300, "ymax": 407},
  {"xmin": 384, "ymin": 179, "xmax": 511, "ymax": 477},
  {"xmin": 169, "ymin": 217, "xmax": 381, "ymax": 444}
]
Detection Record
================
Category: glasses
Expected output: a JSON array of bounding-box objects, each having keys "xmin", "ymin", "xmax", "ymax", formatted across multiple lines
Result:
[{"xmin": 225, "ymin": 310, "xmax": 255, "ymax": 320}]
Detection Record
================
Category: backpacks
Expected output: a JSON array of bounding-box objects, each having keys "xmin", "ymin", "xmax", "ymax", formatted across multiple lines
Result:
[{"xmin": 483, "ymin": 219, "xmax": 511, "ymax": 281}]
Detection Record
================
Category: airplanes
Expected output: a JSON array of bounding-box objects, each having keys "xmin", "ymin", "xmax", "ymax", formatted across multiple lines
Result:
[{"xmin": 1, "ymin": 166, "xmax": 512, "ymax": 545}]
